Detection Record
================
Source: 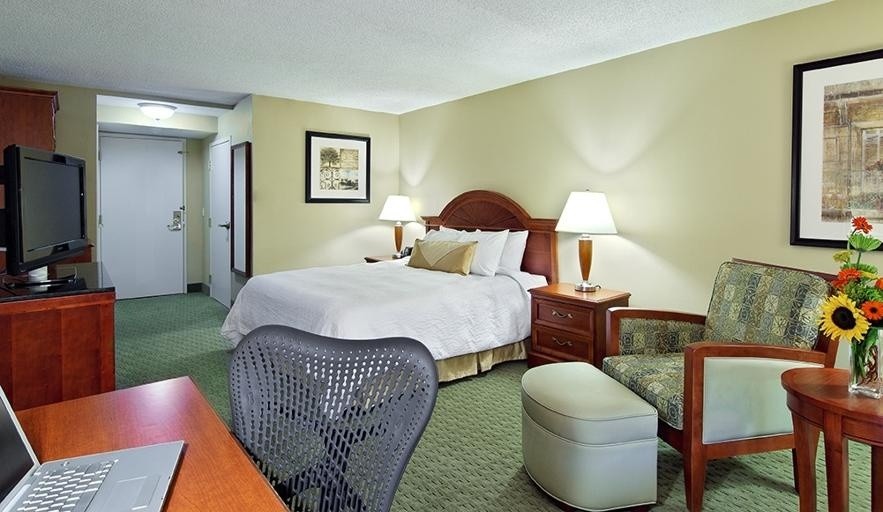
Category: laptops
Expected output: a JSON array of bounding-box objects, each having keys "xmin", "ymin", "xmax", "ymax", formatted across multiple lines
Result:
[{"xmin": 0, "ymin": 386, "xmax": 185, "ymax": 512}]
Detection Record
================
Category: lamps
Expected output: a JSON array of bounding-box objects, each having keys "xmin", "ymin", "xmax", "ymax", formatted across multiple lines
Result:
[
  {"xmin": 135, "ymin": 100, "xmax": 178, "ymax": 127},
  {"xmin": 378, "ymin": 194, "xmax": 418, "ymax": 258},
  {"xmin": 555, "ymin": 189, "xmax": 620, "ymax": 294}
]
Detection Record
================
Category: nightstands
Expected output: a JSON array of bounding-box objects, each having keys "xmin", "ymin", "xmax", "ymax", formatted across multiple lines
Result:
[
  {"xmin": 526, "ymin": 282, "xmax": 631, "ymax": 373},
  {"xmin": 363, "ymin": 254, "xmax": 391, "ymax": 263}
]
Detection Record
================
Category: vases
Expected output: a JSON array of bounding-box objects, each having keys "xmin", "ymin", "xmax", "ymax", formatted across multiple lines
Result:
[{"xmin": 846, "ymin": 324, "xmax": 882, "ymax": 401}]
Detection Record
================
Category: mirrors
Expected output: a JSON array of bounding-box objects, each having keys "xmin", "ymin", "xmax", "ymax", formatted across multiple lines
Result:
[{"xmin": 228, "ymin": 141, "xmax": 252, "ymax": 280}]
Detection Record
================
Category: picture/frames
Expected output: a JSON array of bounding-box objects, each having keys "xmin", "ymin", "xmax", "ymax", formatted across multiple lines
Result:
[
  {"xmin": 302, "ymin": 130, "xmax": 372, "ymax": 206},
  {"xmin": 783, "ymin": 48, "xmax": 882, "ymax": 250}
]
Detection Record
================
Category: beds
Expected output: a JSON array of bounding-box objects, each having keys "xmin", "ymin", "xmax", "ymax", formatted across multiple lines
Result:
[{"xmin": 219, "ymin": 189, "xmax": 561, "ymax": 425}]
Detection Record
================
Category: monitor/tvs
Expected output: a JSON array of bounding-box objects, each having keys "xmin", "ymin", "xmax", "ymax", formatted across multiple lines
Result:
[{"xmin": 0, "ymin": 143, "xmax": 87, "ymax": 288}]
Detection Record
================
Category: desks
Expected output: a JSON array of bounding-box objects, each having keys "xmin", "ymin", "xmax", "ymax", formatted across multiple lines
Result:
[
  {"xmin": 774, "ymin": 367, "xmax": 882, "ymax": 511},
  {"xmin": 9, "ymin": 370, "xmax": 292, "ymax": 512}
]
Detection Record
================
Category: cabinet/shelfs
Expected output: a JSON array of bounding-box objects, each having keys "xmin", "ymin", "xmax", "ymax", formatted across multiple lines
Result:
[
  {"xmin": 2, "ymin": 83, "xmax": 98, "ymax": 265},
  {"xmin": 1, "ymin": 257, "xmax": 121, "ymax": 413}
]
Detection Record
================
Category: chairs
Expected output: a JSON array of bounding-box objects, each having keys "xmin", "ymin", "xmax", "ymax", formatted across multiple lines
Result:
[
  {"xmin": 594, "ymin": 253, "xmax": 852, "ymax": 512},
  {"xmin": 517, "ymin": 358, "xmax": 662, "ymax": 512},
  {"xmin": 225, "ymin": 320, "xmax": 443, "ymax": 511}
]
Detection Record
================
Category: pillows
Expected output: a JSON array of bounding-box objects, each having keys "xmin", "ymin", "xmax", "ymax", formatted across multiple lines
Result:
[
  {"xmin": 421, "ymin": 228, "xmax": 510, "ymax": 280},
  {"xmin": 404, "ymin": 237, "xmax": 479, "ymax": 277},
  {"xmin": 438, "ymin": 225, "xmax": 529, "ymax": 278}
]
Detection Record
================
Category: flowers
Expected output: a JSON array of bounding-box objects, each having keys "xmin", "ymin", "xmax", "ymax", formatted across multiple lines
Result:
[{"xmin": 818, "ymin": 210, "xmax": 882, "ymax": 390}]
